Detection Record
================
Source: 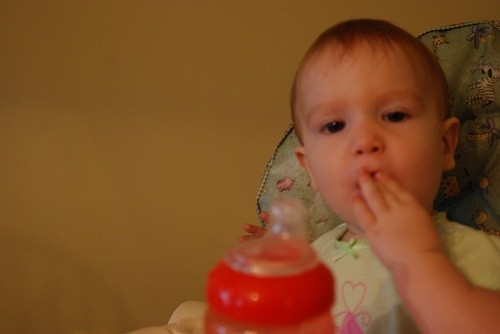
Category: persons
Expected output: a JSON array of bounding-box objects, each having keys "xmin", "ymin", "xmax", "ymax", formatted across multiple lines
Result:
[{"xmin": 119, "ymin": 18, "xmax": 500, "ymax": 334}]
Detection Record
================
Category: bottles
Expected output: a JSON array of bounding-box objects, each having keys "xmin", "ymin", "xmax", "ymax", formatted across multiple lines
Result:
[{"xmin": 203, "ymin": 196, "xmax": 336, "ymax": 334}]
